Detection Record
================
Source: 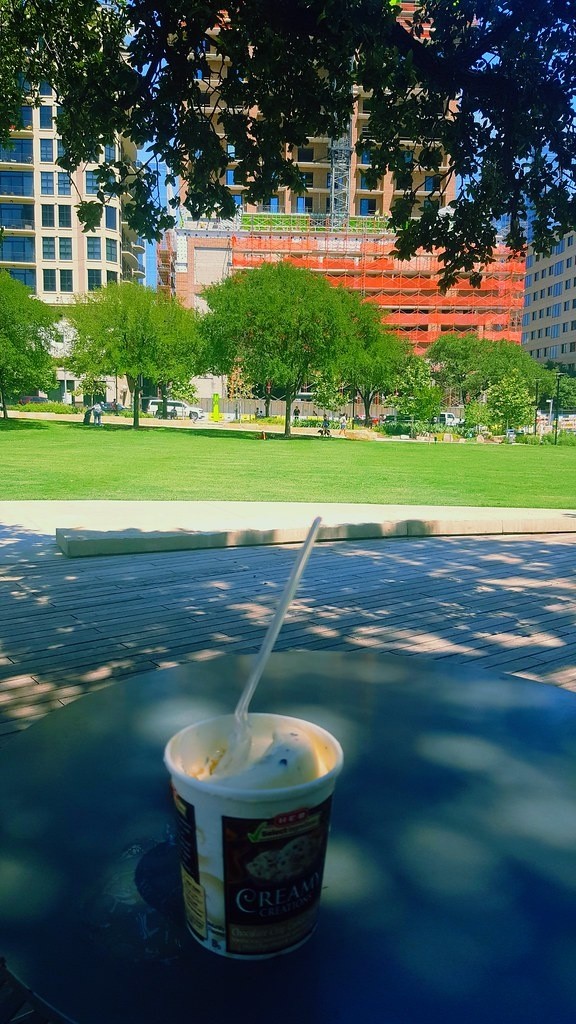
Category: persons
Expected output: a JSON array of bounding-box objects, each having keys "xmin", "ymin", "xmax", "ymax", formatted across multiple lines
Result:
[
  {"xmin": 111, "ymin": 399, "xmax": 117, "ymax": 413},
  {"xmin": 338, "ymin": 413, "xmax": 346, "ymax": 435},
  {"xmin": 171, "ymin": 407, "xmax": 177, "ymax": 419},
  {"xmin": 92, "ymin": 402, "xmax": 101, "ymax": 424},
  {"xmin": 254, "ymin": 407, "xmax": 263, "ymax": 417},
  {"xmin": 321, "ymin": 414, "xmax": 332, "ymax": 436},
  {"xmin": 293, "ymin": 406, "xmax": 300, "ymax": 424}
]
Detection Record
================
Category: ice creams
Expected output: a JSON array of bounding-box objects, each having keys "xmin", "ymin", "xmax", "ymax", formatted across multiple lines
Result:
[
  {"xmin": 244, "ymin": 835, "xmax": 316, "ymax": 892},
  {"xmin": 164, "ymin": 711, "xmax": 344, "ymax": 959}
]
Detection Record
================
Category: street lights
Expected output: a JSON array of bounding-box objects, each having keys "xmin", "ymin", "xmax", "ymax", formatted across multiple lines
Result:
[
  {"xmin": 533, "ymin": 376, "xmax": 542, "ymax": 435},
  {"xmin": 553, "ymin": 372, "xmax": 567, "ymax": 445},
  {"xmin": 546, "ymin": 399, "xmax": 554, "ymax": 426}
]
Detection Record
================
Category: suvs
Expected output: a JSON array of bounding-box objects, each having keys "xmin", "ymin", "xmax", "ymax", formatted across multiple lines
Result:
[{"xmin": 146, "ymin": 400, "xmax": 205, "ymax": 421}]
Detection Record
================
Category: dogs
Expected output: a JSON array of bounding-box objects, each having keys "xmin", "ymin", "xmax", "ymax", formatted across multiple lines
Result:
[{"xmin": 318, "ymin": 430, "xmax": 330, "ymax": 436}]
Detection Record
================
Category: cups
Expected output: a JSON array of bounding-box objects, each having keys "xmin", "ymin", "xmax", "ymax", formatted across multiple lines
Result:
[{"xmin": 164, "ymin": 713, "xmax": 344, "ymax": 959}]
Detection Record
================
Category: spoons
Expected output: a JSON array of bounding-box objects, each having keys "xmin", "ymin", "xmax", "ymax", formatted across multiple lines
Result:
[{"xmin": 211, "ymin": 517, "xmax": 322, "ymax": 774}]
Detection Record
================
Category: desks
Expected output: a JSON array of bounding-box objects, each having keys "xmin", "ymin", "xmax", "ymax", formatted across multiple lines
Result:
[{"xmin": 0, "ymin": 650, "xmax": 576, "ymax": 1024}]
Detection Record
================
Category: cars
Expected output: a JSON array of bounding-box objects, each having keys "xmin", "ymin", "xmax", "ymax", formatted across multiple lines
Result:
[
  {"xmin": 433, "ymin": 412, "xmax": 466, "ymax": 428},
  {"xmin": 101, "ymin": 402, "xmax": 129, "ymax": 412},
  {"xmin": 19, "ymin": 395, "xmax": 48, "ymax": 406}
]
